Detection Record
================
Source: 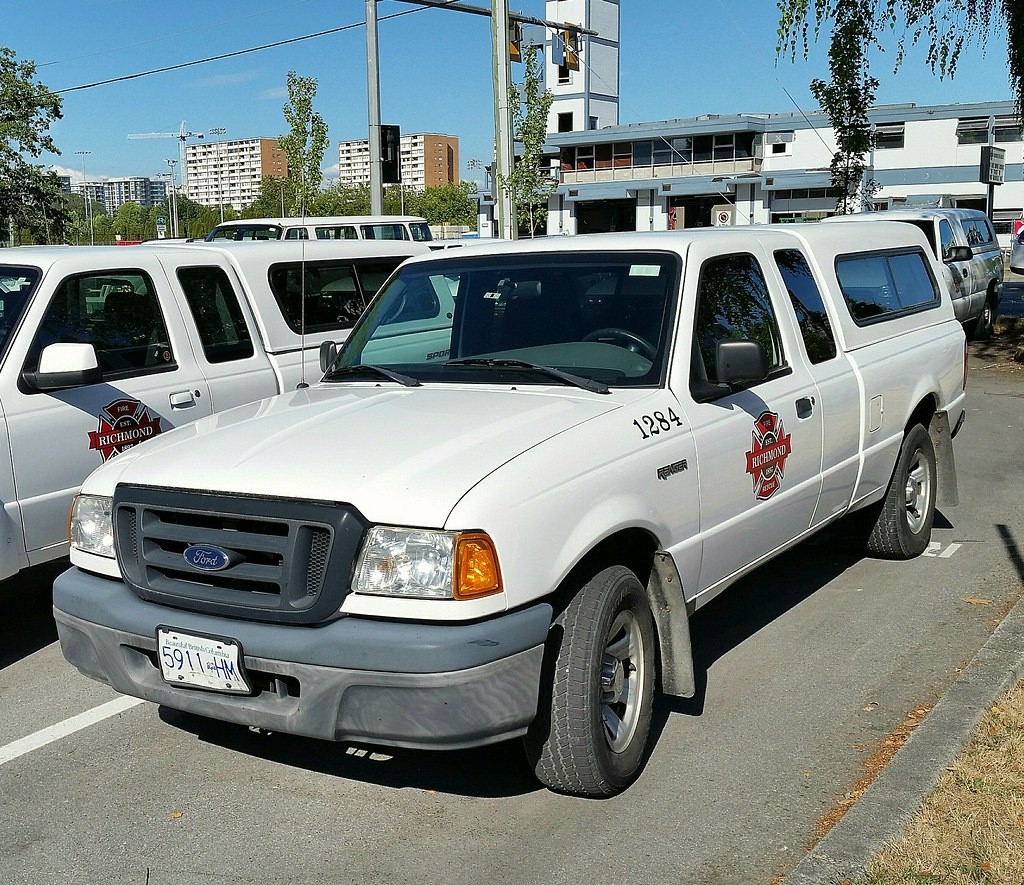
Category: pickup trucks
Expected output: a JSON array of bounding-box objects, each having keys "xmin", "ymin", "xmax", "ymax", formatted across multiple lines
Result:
[
  {"xmin": 2, "ymin": 240, "xmax": 464, "ymax": 593},
  {"xmin": 49, "ymin": 217, "xmax": 968, "ymax": 802}
]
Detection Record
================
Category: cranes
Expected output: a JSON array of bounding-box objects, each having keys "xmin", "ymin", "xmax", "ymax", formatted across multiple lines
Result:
[{"xmin": 125, "ymin": 121, "xmax": 203, "ymax": 198}]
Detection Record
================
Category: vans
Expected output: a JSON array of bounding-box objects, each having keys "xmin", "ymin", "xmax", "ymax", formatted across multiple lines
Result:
[
  {"xmin": 139, "ymin": 209, "xmax": 501, "ymax": 253},
  {"xmin": 819, "ymin": 206, "xmax": 1006, "ymax": 340}
]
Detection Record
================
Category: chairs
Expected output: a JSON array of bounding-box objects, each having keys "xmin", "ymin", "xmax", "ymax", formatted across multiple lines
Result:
[
  {"xmin": 468, "ymin": 278, "xmax": 655, "ymax": 362},
  {"xmin": 90, "ymin": 292, "xmax": 159, "ymax": 370}
]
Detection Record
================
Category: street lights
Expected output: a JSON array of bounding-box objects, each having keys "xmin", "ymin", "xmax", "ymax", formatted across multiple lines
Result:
[
  {"xmin": 210, "ymin": 128, "xmax": 226, "ymax": 224},
  {"xmin": 74, "ymin": 151, "xmax": 91, "ymax": 220}
]
